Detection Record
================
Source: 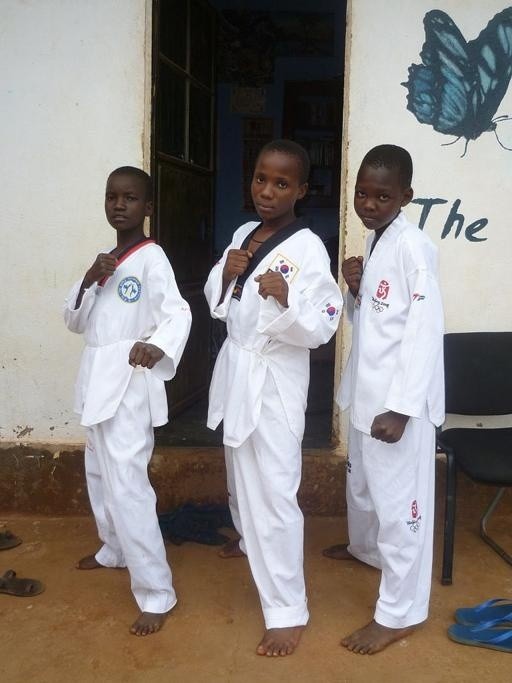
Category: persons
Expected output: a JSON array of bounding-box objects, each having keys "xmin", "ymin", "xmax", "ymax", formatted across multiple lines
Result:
[
  {"xmin": 62, "ymin": 164, "xmax": 194, "ymax": 637},
  {"xmin": 338, "ymin": 141, "xmax": 449, "ymax": 657},
  {"xmin": 201, "ymin": 137, "xmax": 345, "ymax": 659}
]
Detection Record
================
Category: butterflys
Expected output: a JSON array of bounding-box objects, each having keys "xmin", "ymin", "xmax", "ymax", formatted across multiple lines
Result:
[{"xmin": 400, "ymin": 5, "xmax": 512, "ymax": 157}]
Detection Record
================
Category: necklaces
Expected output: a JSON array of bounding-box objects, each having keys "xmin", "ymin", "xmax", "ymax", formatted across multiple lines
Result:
[{"xmin": 251, "ymin": 238, "xmax": 265, "ymax": 245}]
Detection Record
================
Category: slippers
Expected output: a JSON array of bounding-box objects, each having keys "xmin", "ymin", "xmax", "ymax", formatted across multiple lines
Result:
[
  {"xmin": 0, "ymin": 570, "xmax": 44, "ymax": 596},
  {"xmin": 448, "ymin": 597, "xmax": 512, "ymax": 652},
  {"xmin": 0, "ymin": 529, "xmax": 21, "ymax": 550}
]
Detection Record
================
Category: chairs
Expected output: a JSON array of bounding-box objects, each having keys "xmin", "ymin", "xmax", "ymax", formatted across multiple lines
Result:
[{"xmin": 434, "ymin": 332, "xmax": 512, "ymax": 586}]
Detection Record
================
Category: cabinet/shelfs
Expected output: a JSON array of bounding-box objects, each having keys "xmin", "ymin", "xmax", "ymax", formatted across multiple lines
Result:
[
  {"xmin": 284, "ymin": 79, "xmax": 343, "ymax": 200},
  {"xmin": 153, "ymin": 0, "xmax": 217, "ymax": 283}
]
